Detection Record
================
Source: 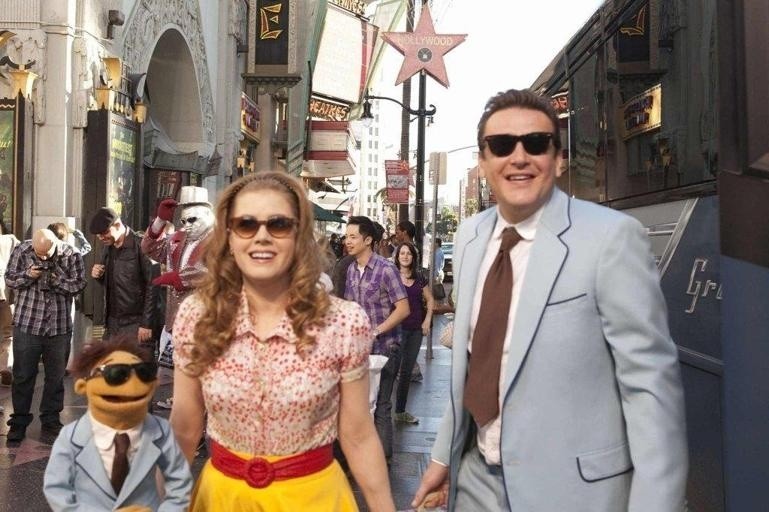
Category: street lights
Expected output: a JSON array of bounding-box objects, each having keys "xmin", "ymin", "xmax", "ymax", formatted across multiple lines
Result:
[
  {"xmin": 409, "ymin": 143, "xmax": 479, "ymax": 172},
  {"xmin": 358, "ymin": 87, "xmax": 436, "ymax": 271}
]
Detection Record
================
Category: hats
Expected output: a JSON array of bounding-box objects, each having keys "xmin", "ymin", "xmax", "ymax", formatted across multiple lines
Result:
[
  {"xmin": 89, "ymin": 207, "xmax": 117, "ymax": 234},
  {"xmin": 176, "ymin": 186, "xmax": 213, "ymax": 209}
]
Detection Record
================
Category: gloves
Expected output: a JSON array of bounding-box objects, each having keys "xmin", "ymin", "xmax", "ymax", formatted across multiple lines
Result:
[
  {"xmin": 152, "ymin": 272, "xmax": 174, "ymax": 286},
  {"xmin": 157, "ymin": 199, "xmax": 177, "ymax": 221}
]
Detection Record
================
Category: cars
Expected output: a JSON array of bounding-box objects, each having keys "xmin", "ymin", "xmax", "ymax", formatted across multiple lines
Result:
[{"xmin": 441, "ymin": 242, "xmax": 455, "ymax": 282}]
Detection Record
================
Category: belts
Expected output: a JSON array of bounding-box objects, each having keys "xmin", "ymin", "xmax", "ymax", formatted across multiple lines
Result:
[{"xmin": 479, "ymin": 452, "xmax": 502, "ymax": 475}]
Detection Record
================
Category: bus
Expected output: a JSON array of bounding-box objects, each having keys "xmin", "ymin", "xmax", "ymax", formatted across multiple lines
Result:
[{"xmin": 476, "ymin": 1, "xmax": 769, "ymax": 511}]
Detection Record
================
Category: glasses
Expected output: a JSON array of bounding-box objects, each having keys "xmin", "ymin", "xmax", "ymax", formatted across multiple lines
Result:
[
  {"xmin": 181, "ymin": 217, "xmax": 196, "ymax": 224},
  {"xmin": 227, "ymin": 215, "xmax": 299, "ymax": 238},
  {"xmin": 484, "ymin": 133, "xmax": 552, "ymax": 156}
]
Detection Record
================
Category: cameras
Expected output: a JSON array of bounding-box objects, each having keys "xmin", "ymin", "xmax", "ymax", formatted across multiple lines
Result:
[{"xmin": 35, "ymin": 261, "xmax": 56, "ymax": 292}]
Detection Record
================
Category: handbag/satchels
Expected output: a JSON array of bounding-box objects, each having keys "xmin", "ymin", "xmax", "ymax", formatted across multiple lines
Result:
[{"xmin": 432, "ymin": 284, "xmax": 445, "ymax": 300}]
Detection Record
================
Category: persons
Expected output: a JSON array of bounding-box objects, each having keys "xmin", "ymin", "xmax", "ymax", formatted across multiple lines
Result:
[
  {"xmin": 1, "ymin": 215, "xmax": 92, "ymax": 442},
  {"xmin": 91, "ymin": 207, "xmax": 167, "ymax": 353},
  {"xmin": 41, "ymin": 331, "xmax": 193, "ymax": 512},
  {"xmin": 155, "ymin": 169, "xmax": 397, "ymax": 512},
  {"xmin": 318, "ymin": 215, "xmax": 453, "ymax": 481},
  {"xmin": 410, "ymin": 90, "xmax": 689, "ymax": 512},
  {"xmin": 140, "ymin": 183, "xmax": 224, "ymax": 411}
]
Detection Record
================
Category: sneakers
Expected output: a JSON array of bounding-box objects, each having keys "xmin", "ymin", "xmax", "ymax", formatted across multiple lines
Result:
[
  {"xmin": 157, "ymin": 398, "xmax": 173, "ymax": 408},
  {"xmin": 0, "ymin": 371, "xmax": 12, "ymax": 387},
  {"xmin": 7, "ymin": 425, "xmax": 26, "ymax": 441},
  {"xmin": 396, "ymin": 371, "xmax": 423, "ymax": 382},
  {"xmin": 394, "ymin": 412, "xmax": 418, "ymax": 423},
  {"xmin": 41, "ymin": 420, "xmax": 63, "ymax": 435}
]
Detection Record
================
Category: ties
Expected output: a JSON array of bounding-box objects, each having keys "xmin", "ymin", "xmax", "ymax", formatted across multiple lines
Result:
[{"xmin": 463, "ymin": 227, "xmax": 521, "ymax": 429}]
